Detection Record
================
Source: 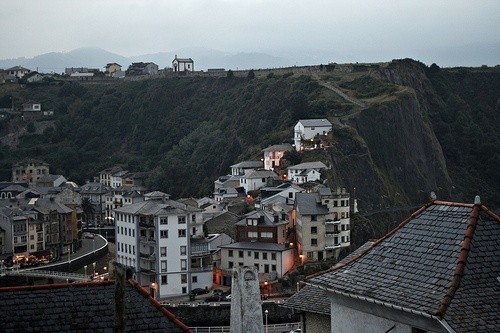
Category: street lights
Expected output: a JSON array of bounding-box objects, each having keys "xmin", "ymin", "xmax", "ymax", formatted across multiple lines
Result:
[{"xmin": 264, "ymin": 281, "xmax": 270, "ymax": 299}]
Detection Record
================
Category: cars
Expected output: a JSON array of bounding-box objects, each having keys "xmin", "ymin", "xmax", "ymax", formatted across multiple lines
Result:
[
  {"xmin": 191, "ymin": 288, "xmax": 209, "ymax": 295},
  {"xmin": 225, "ymin": 295, "xmax": 231, "ymax": 301},
  {"xmin": 85, "ymin": 233, "xmax": 94, "ymax": 239},
  {"xmin": 204, "ymin": 294, "xmax": 223, "ymax": 302},
  {"xmin": 88, "ymin": 223, "xmax": 104, "ymax": 228},
  {"xmin": 1, "ymin": 252, "xmax": 64, "ymax": 273}
]
